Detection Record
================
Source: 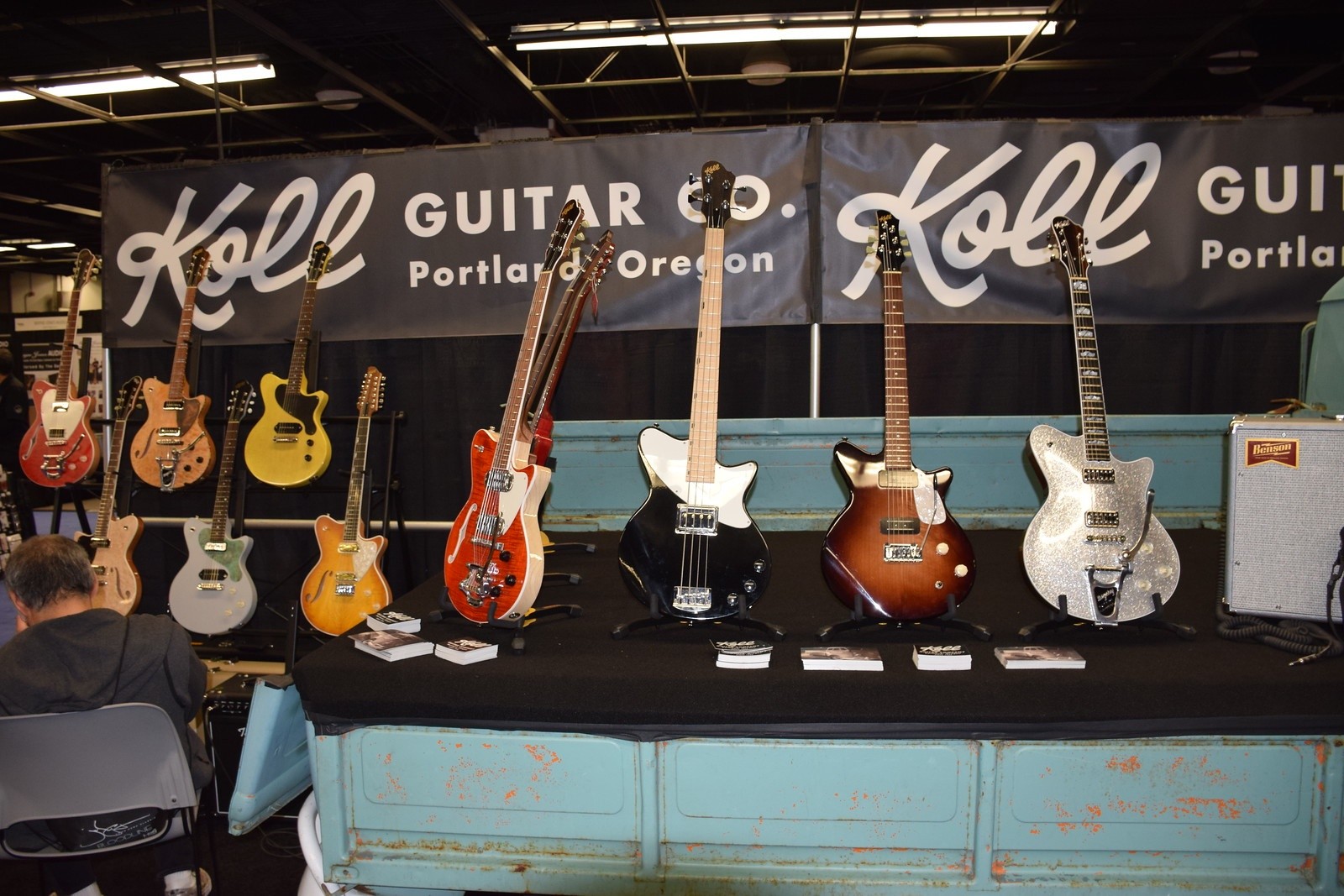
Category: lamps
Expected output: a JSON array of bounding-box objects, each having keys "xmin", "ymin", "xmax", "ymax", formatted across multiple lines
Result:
[
  {"xmin": 315, "ymin": 71, "xmax": 362, "ymax": 109},
  {"xmin": 740, "ymin": 42, "xmax": 792, "ymax": 85},
  {"xmin": 510, "ymin": 5, "xmax": 1058, "ymax": 51},
  {"xmin": 1207, "ymin": 27, "xmax": 1259, "ymax": 74},
  {"xmin": 0, "ymin": 53, "xmax": 276, "ymax": 102}
]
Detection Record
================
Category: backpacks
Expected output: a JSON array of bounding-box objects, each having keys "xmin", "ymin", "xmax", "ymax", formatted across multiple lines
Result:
[{"xmin": 23, "ymin": 807, "xmax": 170, "ymax": 856}]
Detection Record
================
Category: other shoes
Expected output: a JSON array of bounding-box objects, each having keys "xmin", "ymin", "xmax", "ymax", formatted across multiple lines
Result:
[{"xmin": 163, "ymin": 867, "xmax": 212, "ymax": 896}]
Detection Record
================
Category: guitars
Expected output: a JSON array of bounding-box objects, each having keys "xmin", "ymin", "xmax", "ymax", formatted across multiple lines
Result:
[
  {"xmin": 1018, "ymin": 213, "xmax": 1182, "ymax": 632},
  {"xmin": 616, "ymin": 159, "xmax": 775, "ymax": 628},
  {"xmin": 299, "ymin": 365, "xmax": 394, "ymax": 645},
  {"xmin": 129, "ymin": 245, "xmax": 218, "ymax": 492},
  {"xmin": 817, "ymin": 206, "xmax": 980, "ymax": 625},
  {"xmin": 18, "ymin": 247, "xmax": 102, "ymax": 491},
  {"xmin": 72, "ymin": 374, "xmax": 143, "ymax": 617},
  {"xmin": 440, "ymin": 196, "xmax": 588, "ymax": 625},
  {"xmin": 167, "ymin": 379, "xmax": 260, "ymax": 637},
  {"xmin": 243, "ymin": 240, "xmax": 334, "ymax": 492},
  {"xmin": 527, "ymin": 228, "xmax": 617, "ymax": 466}
]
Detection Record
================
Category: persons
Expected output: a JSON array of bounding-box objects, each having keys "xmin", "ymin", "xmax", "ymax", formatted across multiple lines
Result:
[{"xmin": 0, "ymin": 534, "xmax": 212, "ymax": 896}]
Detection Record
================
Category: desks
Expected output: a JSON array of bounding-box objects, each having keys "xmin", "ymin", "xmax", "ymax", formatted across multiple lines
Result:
[{"xmin": 291, "ymin": 413, "xmax": 1344, "ymax": 896}]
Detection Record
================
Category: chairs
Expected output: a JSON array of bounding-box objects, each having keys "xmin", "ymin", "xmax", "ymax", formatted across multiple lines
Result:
[{"xmin": 0, "ymin": 702, "xmax": 217, "ymax": 896}]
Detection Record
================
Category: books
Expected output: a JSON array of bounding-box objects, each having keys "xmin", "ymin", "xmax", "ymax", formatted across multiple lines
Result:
[
  {"xmin": 709, "ymin": 637, "xmax": 773, "ymax": 669},
  {"xmin": 347, "ymin": 610, "xmax": 500, "ymax": 666},
  {"xmin": 800, "ymin": 642, "xmax": 1088, "ymax": 672}
]
